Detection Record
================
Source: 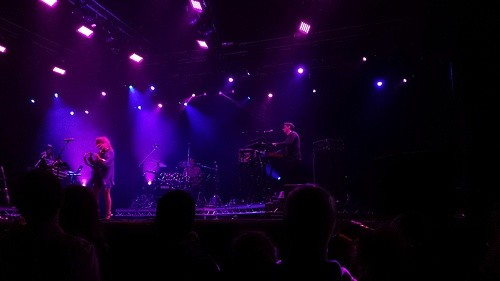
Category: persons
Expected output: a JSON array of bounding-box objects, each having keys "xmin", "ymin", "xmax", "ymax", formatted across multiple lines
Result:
[
  {"xmin": 0, "ymin": 183, "xmax": 500, "ymax": 281},
  {"xmin": 93, "ymin": 137, "xmax": 115, "ymax": 219},
  {"xmin": 34, "ymin": 143, "xmax": 56, "ymax": 168},
  {"xmin": 272, "ymin": 122, "xmax": 301, "ymax": 184}
]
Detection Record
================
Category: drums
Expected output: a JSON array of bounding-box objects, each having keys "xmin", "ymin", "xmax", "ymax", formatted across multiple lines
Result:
[{"xmin": 237, "ymin": 148, "xmax": 259, "ymax": 164}]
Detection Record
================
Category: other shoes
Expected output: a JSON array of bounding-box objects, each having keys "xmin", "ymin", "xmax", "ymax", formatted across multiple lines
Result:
[{"xmin": 103, "ymin": 213, "xmax": 113, "ymax": 220}]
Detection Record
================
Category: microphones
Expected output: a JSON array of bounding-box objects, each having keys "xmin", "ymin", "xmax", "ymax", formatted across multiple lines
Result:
[
  {"xmin": 264, "ymin": 129, "xmax": 274, "ymax": 133},
  {"xmin": 64, "ymin": 137, "xmax": 75, "ymax": 141}
]
